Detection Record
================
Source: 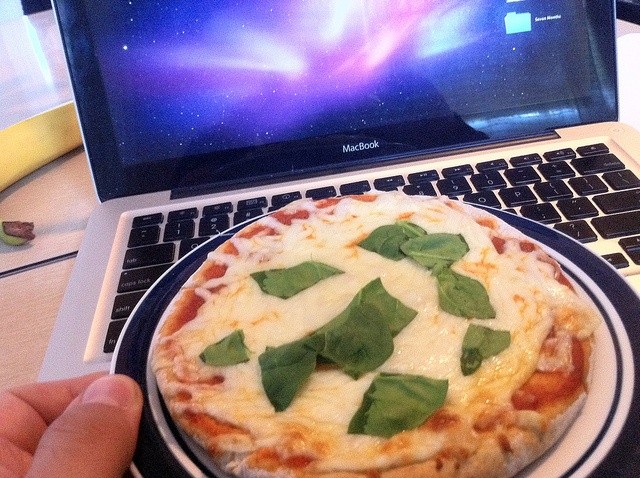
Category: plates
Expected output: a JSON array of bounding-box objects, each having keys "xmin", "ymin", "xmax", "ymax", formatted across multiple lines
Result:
[{"xmin": 109, "ymin": 198, "xmax": 640, "ymax": 477}]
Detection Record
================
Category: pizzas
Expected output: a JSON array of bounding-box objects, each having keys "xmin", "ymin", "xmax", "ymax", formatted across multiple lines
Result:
[{"xmin": 150, "ymin": 191, "xmax": 602, "ymax": 478}]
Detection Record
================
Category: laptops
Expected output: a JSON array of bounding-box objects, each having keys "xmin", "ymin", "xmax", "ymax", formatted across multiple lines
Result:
[{"xmin": 36, "ymin": 0, "xmax": 638, "ymax": 388}]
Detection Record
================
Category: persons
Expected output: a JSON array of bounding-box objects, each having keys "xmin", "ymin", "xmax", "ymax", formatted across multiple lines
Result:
[{"xmin": 0, "ymin": 371, "xmax": 144, "ymax": 478}]
